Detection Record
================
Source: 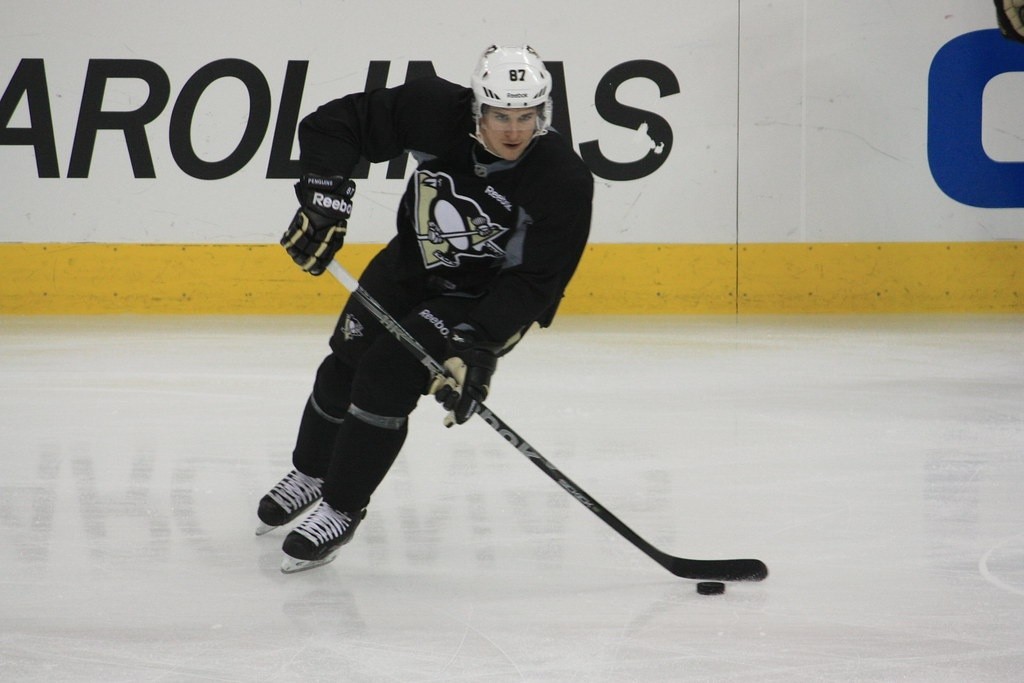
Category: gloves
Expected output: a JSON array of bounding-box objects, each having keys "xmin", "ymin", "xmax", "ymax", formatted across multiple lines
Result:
[
  {"xmin": 281, "ymin": 174, "xmax": 357, "ymax": 276},
  {"xmin": 431, "ymin": 324, "xmax": 496, "ymax": 428}
]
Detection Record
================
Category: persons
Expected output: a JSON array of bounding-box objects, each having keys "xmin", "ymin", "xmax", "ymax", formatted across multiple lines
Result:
[{"xmin": 255, "ymin": 45, "xmax": 594, "ymax": 574}]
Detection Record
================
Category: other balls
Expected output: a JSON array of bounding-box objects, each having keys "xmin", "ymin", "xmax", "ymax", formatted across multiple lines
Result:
[{"xmin": 696, "ymin": 581, "xmax": 726, "ymax": 595}]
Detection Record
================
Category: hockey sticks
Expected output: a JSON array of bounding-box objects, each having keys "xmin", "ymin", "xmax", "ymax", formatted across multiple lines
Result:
[{"xmin": 327, "ymin": 259, "xmax": 770, "ymax": 583}]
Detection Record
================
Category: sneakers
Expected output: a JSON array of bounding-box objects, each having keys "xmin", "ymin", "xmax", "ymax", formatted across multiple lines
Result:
[
  {"xmin": 280, "ymin": 500, "xmax": 368, "ymax": 573},
  {"xmin": 256, "ymin": 466, "xmax": 325, "ymax": 536}
]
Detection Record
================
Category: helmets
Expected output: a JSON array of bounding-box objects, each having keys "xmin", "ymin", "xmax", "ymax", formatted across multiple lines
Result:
[{"xmin": 473, "ymin": 44, "xmax": 554, "ymax": 132}]
